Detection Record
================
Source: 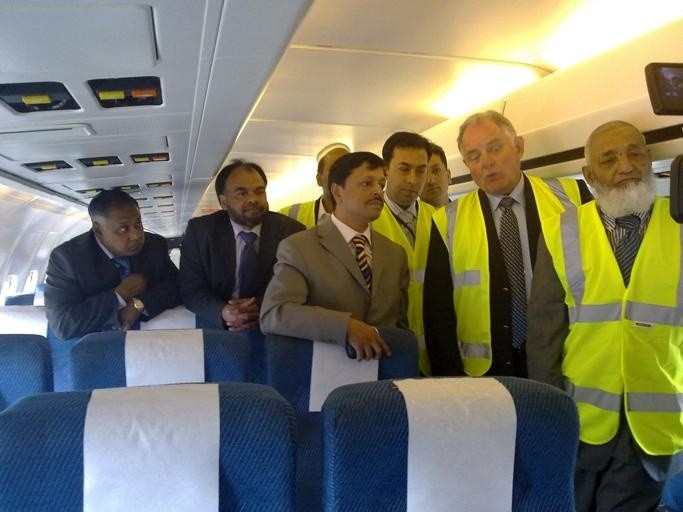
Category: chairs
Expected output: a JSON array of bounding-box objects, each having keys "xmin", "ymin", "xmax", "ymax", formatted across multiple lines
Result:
[
  {"xmin": 128, "ymin": 304, "xmax": 216, "ymax": 330},
  {"xmin": 69, "ymin": 329, "xmax": 258, "ymax": 389},
  {"xmin": 321, "ymin": 376, "xmax": 579, "ymax": 511},
  {"xmin": 1, "ymin": 383, "xmax": 297, "ymax": 512},
  {"xmin": 35, "ymin": 284, "xmax": 45, "ymax": 293},
  {"xmin": 260, "ymin": 326, "xmax": 420, "ymax": 512},
  {"xmin": 0, "ymin": 333, "xmax": 53, "ymax": 412},
  {"xmin": 0, "ymin": 306, "xmax": 71, "ymax": 392},
  {"xmin": 5, "ymin": 293, "xmax": 45, "ymax": 306}
]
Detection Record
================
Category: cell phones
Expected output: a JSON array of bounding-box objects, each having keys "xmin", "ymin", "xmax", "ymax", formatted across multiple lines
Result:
[{"xmin": 344, "ymin": 322, "xmax": 384, "ymax": 359}]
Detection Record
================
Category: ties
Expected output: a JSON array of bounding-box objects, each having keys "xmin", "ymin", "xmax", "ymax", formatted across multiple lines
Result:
[
  {"xmin": 352, "ymin": 236, "xmax": 372, "ymax": 290},
  {"xmin": 111, "ymin": 256, "xmax": 140, "ymax": 331},
  {"xmin": 499, "ymin": 197, "xmax": 528, "ymax": 348},
  {"xmin": 239, "ymin": 232, "xmax": 258, "ymax": 298},
  {"xmin": 615, "ymin": 214, "xmax": 642, "ymax": 288},
  {"xmin": 398, "ymin": 211, "xmax": 416, "ymax": 248}
]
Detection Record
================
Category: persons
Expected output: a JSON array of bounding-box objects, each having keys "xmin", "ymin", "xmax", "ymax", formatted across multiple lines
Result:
[
  {"xmin": 375, "ymin": 133, "xmax": 449, "ymax": 376},
  {"xmin": 451, "ymin": 111, "xmax": 593, "ymax": 378},
  {"xmin": 421, "ymin": 143, "xmax": 452, "ymax": 207},
  {"xmin": 279, "ymin": 148, "xmax": 346, "ymax": 230},
  {"xmin": 259, "ymin": 152, "xmax": 408, "ymax": 363},
  {"xmin": 527, "ymin": 122, "xmax": 681, "ymax": 512},
  {"xmin": 42, "ymin": 190, "xmax": 181, "ymax": 341},
  {"xmin": 180, "ymin": 158, "xmax": 306, "ymax": 328}
]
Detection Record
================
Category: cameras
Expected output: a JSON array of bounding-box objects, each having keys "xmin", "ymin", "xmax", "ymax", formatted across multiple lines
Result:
[{"xmin": 645, "ymin": 62, "xmax": 683, "ymax": 116}]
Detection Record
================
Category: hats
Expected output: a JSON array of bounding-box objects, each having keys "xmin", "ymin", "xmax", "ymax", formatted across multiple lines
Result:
[{"xmin": 315, "ymin": 142, "xmax": 352, "ymax": 162}]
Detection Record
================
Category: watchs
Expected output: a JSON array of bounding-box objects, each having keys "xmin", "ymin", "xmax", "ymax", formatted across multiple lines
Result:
[{"xmin": 130, "ymin": 297, "xmax": 145, "ymax": 312}]
